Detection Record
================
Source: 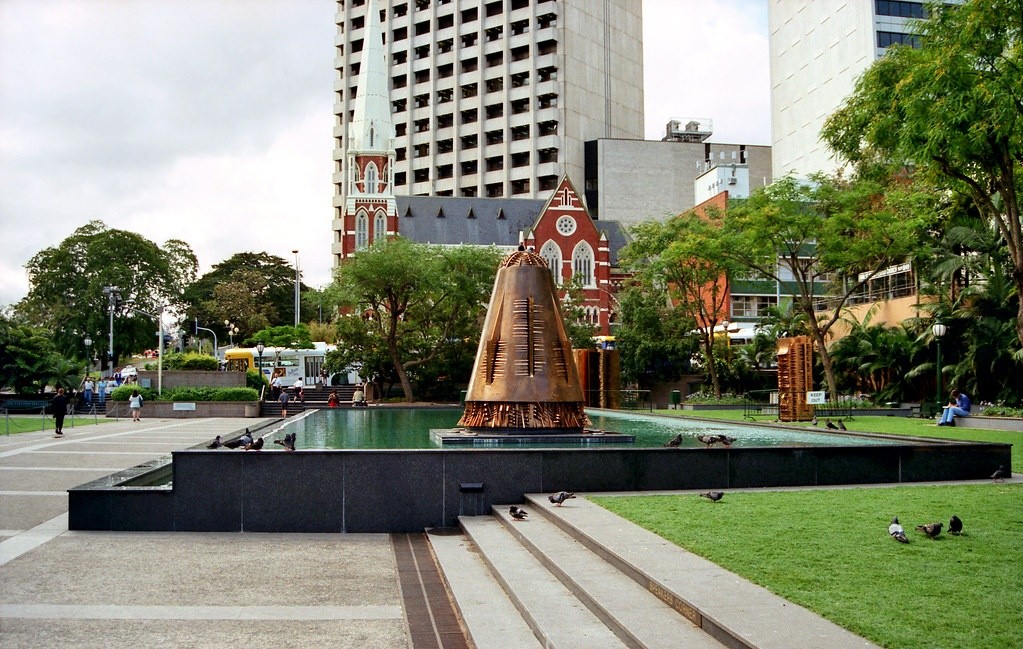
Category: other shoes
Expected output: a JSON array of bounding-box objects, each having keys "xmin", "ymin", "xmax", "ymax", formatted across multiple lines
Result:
[
  {"xmin": 134, "ymin": 420, "xmax": 135, "ymax": 421},
  {"xmin": 55, "ymin": 429, "xmax": 63, "ymax": 434},
  {"xmin": 137, "ymin": 419, "xmax": 140, "ymax": 421}
]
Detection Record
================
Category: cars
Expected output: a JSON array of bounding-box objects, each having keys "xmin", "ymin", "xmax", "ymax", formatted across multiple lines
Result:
[{"xmin": 113, "ymin": 364, "xmax": 139, "ymax": 379}]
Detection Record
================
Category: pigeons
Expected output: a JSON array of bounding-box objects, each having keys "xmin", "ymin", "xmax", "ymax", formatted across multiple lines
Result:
[
  {"xmin": 697, "ymin": 435, "xmax": 718, "ymax": 448},
  {"xmin": 699, "ymin": 491, "xmax": 724, "ymax": 502},
  {"xmin": 666, "ymin": 434, "xmax": 682, "ymax": 448},
  {"xmin": 812, "ymin": 416, "xmax": 818, "ymax": 427},
  {"xmin": 508, "ymin": 506, "xmax": 528, "ymax": 520},
  {"xmin": 946, "ymin": 515, "xmax": 963, "ymax": 535},
  {"xmin": 990, "ymin": 465, "xmax": 1006, "ymax": 483},
  {"xmin": 837, "ymin": 419, "xmax": 847, "ymax": 430},
  {"xmin": 236, "ymin": 428, "xmax": 264, "ymax": 450},
  {"xmin": 718, "ymin": 434, "xmax": 737, "ymax": 448},
  {"xmin": 825, "ymin": 417, "xmax": 839, "ymax": 430},
  {"xmin": 888, "ymin": 516, "xmax": 908, "ymax": 543},
  {"xmin": 274, "ymin": 433, "xmax": 296, "ymax": 450},
  {"xmin": 548, "ymin": 491, "xmax": 576, "ymax": 507},
  {"xmin": 915, "ymin": 523, "xmax": 943, "ymax": 540},
  {"xmin": 206, "ymin": 435, "xmax": 221, "ymax": 449}
]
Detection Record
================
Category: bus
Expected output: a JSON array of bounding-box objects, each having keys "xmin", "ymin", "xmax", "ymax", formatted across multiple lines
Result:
[{"xmin": 226, "ymin": 341, "xmax": 376, "ymax": 389}]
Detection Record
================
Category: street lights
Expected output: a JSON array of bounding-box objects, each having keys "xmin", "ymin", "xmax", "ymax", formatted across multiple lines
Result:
[
  {"xmin": 85, "ymin": 334, "xmax": 92, "ymax": 377},
  {"xmin": 225, "ymin": 320, "xmax": 239, "ymax": 348},
  {"xmin": 722, "ymin": 317, "xmax": 729, "ymax": 361},
  {"xmin": 932, "ymin": 314, "xmax": 947, "ymax": 417},
  {"xmin": 257, "ymin": 339, "xmax": 264, "ymax": 396},
  {"xmin": 292, "ymin": 250, "xmax": 300, "ymax": 328}
]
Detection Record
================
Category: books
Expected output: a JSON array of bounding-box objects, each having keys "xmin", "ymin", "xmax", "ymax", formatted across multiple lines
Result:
[{"xmin": 942, "ymin": 406, "xmax": 950, "ymax": 408}]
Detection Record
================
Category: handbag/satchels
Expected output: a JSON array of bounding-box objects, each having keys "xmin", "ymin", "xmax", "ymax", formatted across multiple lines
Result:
[{"xmin": 139, "ymin": 396, "xmax": 143, "ymax": 408}]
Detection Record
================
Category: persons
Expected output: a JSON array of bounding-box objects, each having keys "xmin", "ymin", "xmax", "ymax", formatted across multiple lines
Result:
[
  {"xmin": 107, "ymin": 368, "xmax": 121, "ymax": 387},
  {"xmin": 328, "ymin": 391, "xmax": 340, "ymax": 406},
  {"xmin": 129, "ymin": 390, "xmax": 143, "ymax": 421},
  {"xmin": 938, "ymin": 390, "xmax": 971, "ymax": 427},
  {"xmin": 84, "ymin": 378, "xmax": 94, "ymax": 406},
  {"xmin": 269, "ymin": 373, "xmax": 282, "ymax": 401},
  {"xmin": 97, "ymin": 377, "xmax": 107, "ymax": 405},
  {"xmin": 54, "ymin": 388, "xmax": 69, "ymax": 434},
  {"xmin": 353, "ymin": 388, "xmax": 364, "ymax": 402},
  {"xmin": 278, "ymin": 389, "xmax": 289, "ymax": 418},
  {"xmin": 293, "ymin": 377, "xmax": 304, "ymax": 404}
]
